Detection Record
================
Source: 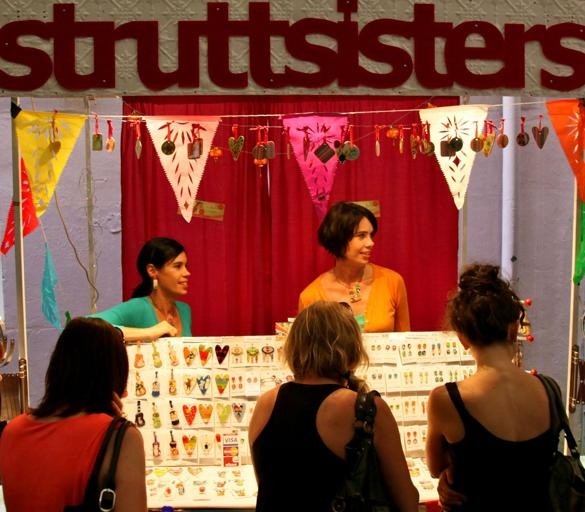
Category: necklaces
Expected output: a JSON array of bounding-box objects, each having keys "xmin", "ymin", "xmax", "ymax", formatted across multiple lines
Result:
[{"xmin": 337, "ymin": 276, "xmax": 365, "ymax": 301}]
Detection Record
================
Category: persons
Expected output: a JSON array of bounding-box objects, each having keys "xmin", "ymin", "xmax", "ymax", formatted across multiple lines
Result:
[
  {"xmin": 426, "ymin": 261, "xmax": 564, "ymax": 512},
  {"xmin": 0, "ymin": 315, "xmax": 143, "ymax": 512},
  {"xmin": 247, "ymin": 298, "xmax": 420, "ymax": 512},
  {"xmin": 299, "ymin": 202, "xmax": 411, "ymax": 332},
  {"xmin": 84, "ymin": 237, "xmax": 193, "ymax": 343}
]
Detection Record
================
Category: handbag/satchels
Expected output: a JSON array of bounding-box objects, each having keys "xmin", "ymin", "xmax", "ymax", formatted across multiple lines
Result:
[
  {"xmin": 327, "ymin": 390, "xmax": 395, "ymax": 512},
  {"xmin": 64, "ymin": 417, "xmax": 130, "ymax": 512},
  {"xmin": 536, "ymin": 374, "xmax": 585, "ymax": 512}
]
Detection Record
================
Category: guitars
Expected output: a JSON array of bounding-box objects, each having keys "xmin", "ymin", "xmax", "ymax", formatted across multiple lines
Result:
[{"xmin": 134, "ymin": 340, "xmax": 180, "ymax": 458}]
